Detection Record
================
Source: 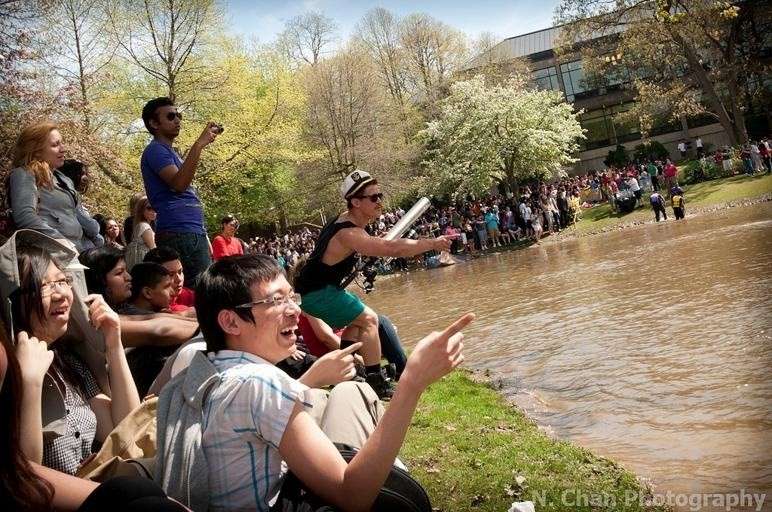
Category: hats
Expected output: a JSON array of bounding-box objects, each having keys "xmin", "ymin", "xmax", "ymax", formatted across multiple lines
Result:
[{"xmin": 340, "ymin": 169, "xmax": 375, "ymax": 199}]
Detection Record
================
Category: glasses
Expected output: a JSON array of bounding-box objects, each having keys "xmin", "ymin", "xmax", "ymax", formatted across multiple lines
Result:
[
  {"xmin": 236, "ymin": 292, "xmax": 302, "ymax": 309},
  {"xmin": 146, "ymin": 205, "xmax": 153, "ymax": 210},
  {"xmin": 357, "ymin": 193, "xmax": 383, "ymax": 202},
  {"xmin": 40, "ymin": 276, "xmax": 74, "ymax": 297},
  {"xmin": 167, "ymin": 112, "xmax": 182, "ymax": 121}
]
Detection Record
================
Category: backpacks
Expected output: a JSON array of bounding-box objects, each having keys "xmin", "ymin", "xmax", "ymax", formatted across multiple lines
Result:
[{"xmin": 275, "ymin": 443, "xmax": 433, "ymax": 512}]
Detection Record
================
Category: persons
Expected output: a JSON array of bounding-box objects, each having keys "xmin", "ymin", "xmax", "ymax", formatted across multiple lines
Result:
[{"xmin": 2, "ymin": 95, "xmax": 772, "ymax": 511}]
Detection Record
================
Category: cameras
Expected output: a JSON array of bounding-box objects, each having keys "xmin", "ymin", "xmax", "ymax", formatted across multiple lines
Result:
[{"xmin": 210, "ymin": 122, "xmax": 225, "ymax": 134}]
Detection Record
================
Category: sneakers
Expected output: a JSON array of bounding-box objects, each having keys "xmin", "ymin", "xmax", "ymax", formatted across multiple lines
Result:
[{"xmin": 370, "ymin": 373, "xmax": 394, "ymax": 402}]
